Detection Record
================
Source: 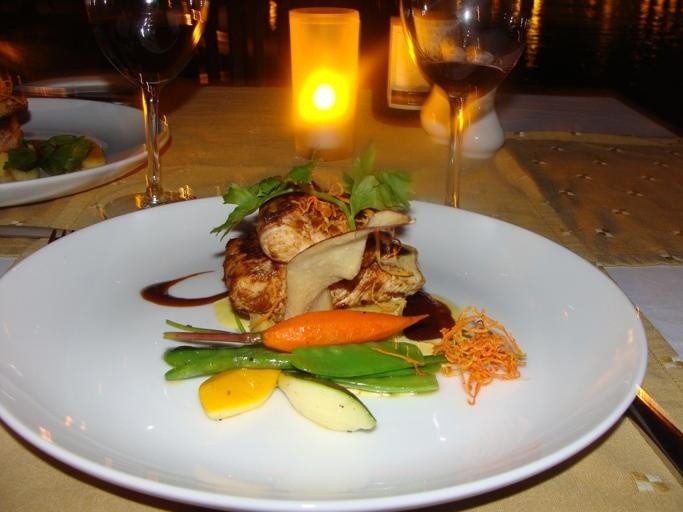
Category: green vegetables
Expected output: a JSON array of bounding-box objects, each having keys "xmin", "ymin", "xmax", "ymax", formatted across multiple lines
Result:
[{"xmin": 210, "ymin": 140, "xmax": 415, "ymax": 241}]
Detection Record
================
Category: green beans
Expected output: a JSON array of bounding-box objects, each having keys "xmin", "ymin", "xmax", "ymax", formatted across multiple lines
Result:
[{"xmin": 162, "ymin": 339, "xmax": 449, "ymax": 393}]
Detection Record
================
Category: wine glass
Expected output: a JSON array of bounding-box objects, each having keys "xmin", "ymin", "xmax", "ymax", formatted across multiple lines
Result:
[
  {"xmin": 84, "ymin": 1, "xmax": 210, "ymax": 220},
  {"xmin": 400, "ymin": 1, "xmax": 533, "ymax": 208}
]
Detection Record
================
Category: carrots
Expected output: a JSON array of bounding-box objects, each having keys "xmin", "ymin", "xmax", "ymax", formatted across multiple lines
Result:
[{"xmin": 260, "ymin": 311, "xmax": 431, "ymax": 353}]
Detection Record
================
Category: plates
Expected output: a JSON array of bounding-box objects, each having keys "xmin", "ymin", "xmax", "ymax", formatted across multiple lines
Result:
[
  {"xmin": 1, "ymin": 188, "xmax": 650, "ymax": 512},
  {"xmin": 0, "ymin": 96, "xmax": 170, "ymax": 215}
]
